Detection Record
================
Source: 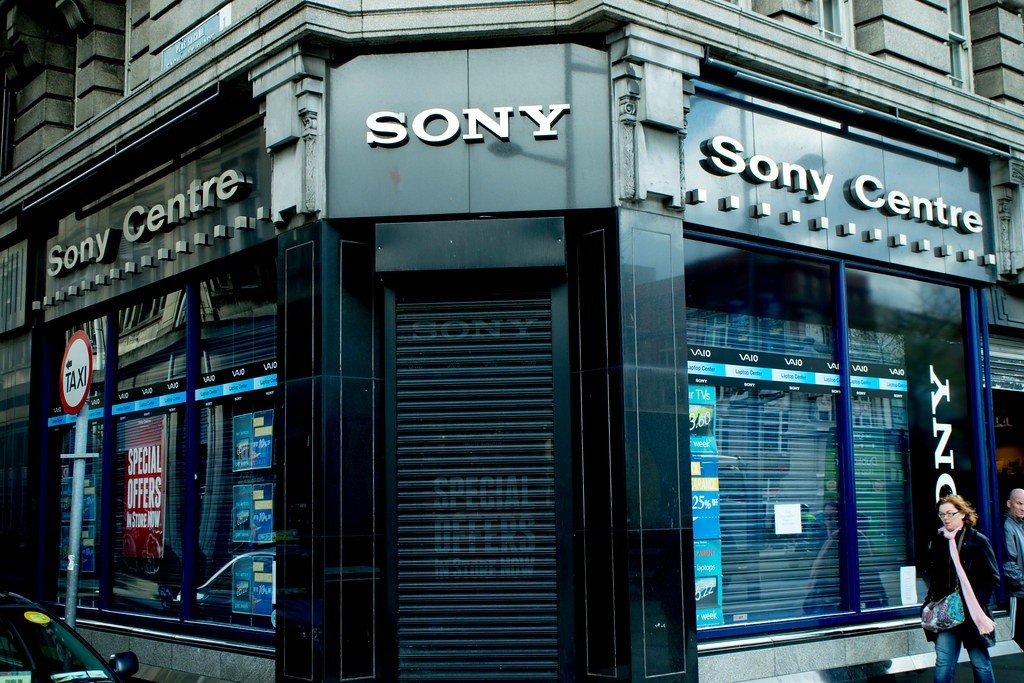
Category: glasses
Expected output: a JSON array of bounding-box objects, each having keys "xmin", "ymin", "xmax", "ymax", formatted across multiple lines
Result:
[{"xmin": 938, "ymin": 510, "xmax": 960, "ymax": 518}]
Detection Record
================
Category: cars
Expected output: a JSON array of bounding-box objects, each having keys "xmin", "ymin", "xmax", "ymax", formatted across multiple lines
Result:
[
  {"xmin": 0, "ymin": 592, "xmax": 156, "ymax": 682},
  {"xmin": 156, "ymin": 551, "xmax": 277, "ymax": 633}
]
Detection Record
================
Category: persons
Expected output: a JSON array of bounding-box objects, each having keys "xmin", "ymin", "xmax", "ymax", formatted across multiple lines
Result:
[
  {"xmin": 1001, "ymin": 488, "xmax": 1023, "ymax": 654},
  {"xmin": 800, "ymin": 502, "xmax": 892, "ymax": 620},
  {"xmin": 918, "ymin": 492, "xmax": 1001, "ymax": 683}
]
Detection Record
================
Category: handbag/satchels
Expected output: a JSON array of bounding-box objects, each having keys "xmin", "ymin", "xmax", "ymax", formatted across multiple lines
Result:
[{"xmin": 921, "ymin": 592, "xmax": 965, "ymax": 631}]
